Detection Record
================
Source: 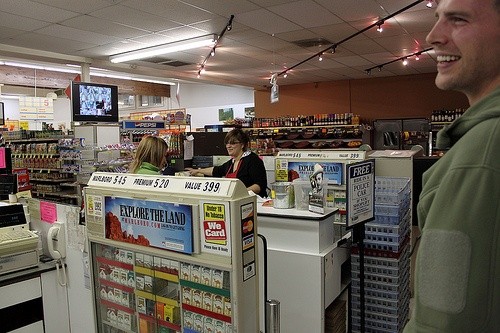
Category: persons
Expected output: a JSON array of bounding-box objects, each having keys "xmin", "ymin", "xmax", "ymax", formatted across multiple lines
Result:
[
  {"xmin": 185, "ymin": 129, "xmax": 267, "ymax": 199},
  {"xmin": 401, "ymin": 0, "xmax": 500, "ymax": 333},
  {"xmin": 131, "ymin": 136, "xmax": 168, "ymax": 175}
]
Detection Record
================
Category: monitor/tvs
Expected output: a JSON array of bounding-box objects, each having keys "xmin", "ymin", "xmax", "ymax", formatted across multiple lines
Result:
[{"xmin": 71, "ymin": 81, "xmax": 118, "ymax": 122}]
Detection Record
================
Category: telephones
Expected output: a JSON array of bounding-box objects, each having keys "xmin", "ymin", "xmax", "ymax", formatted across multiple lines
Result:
[{"xmin": 47, "ymin": 221, "xmax": 66, "ymax": 262}]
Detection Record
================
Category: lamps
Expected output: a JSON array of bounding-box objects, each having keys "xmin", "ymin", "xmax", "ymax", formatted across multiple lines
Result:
[
  {"xmin": 0, "ymin": 15, "xmax": 237, "ymax": 85},
  {"xmin": 271, "ymin": 1, "xmax": 438, "ymax": 82}
]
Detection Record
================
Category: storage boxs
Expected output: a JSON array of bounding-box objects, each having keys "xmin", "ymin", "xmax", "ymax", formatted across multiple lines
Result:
[{"xmin": 345, "ymin": 176, "xmax": 413, "ymax": 332}]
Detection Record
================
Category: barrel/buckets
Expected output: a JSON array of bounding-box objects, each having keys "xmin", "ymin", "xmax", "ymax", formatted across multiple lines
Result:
[{"xmin": 269, "ymin": 182, "xmax": 295, "ymax": 209}]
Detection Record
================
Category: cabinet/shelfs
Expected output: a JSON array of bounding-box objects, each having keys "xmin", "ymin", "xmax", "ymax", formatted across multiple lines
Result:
[
  {"xmin": 250, "ymin": 124, "xmax": 361, "ymax": 150},
  {"xmin": 429, "ymin": 127, "xmax": 453, "ymax": 156},
  {"xmin": 0, "ymin": 116, "xmax": 194, "ymax": 228}
]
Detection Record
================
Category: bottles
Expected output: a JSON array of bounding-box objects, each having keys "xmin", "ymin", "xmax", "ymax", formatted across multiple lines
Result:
[
  {"xmin": 431, "ymin": 108, "xmax": 467, "ymax": 122},
  {"xmin": 231, "ymin": 112, "xmax": 359, "ymax": 155}
]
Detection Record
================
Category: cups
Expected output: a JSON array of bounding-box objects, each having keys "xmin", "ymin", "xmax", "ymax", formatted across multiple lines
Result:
[{"xmin": 9, "ymin": 194, "xmax": 17, "ymax": 202}]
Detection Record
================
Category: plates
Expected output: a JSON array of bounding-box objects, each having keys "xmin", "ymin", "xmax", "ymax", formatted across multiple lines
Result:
[{"xmin": 141, "ymin": 110, "xmax": 185, "ymax": 120}]
